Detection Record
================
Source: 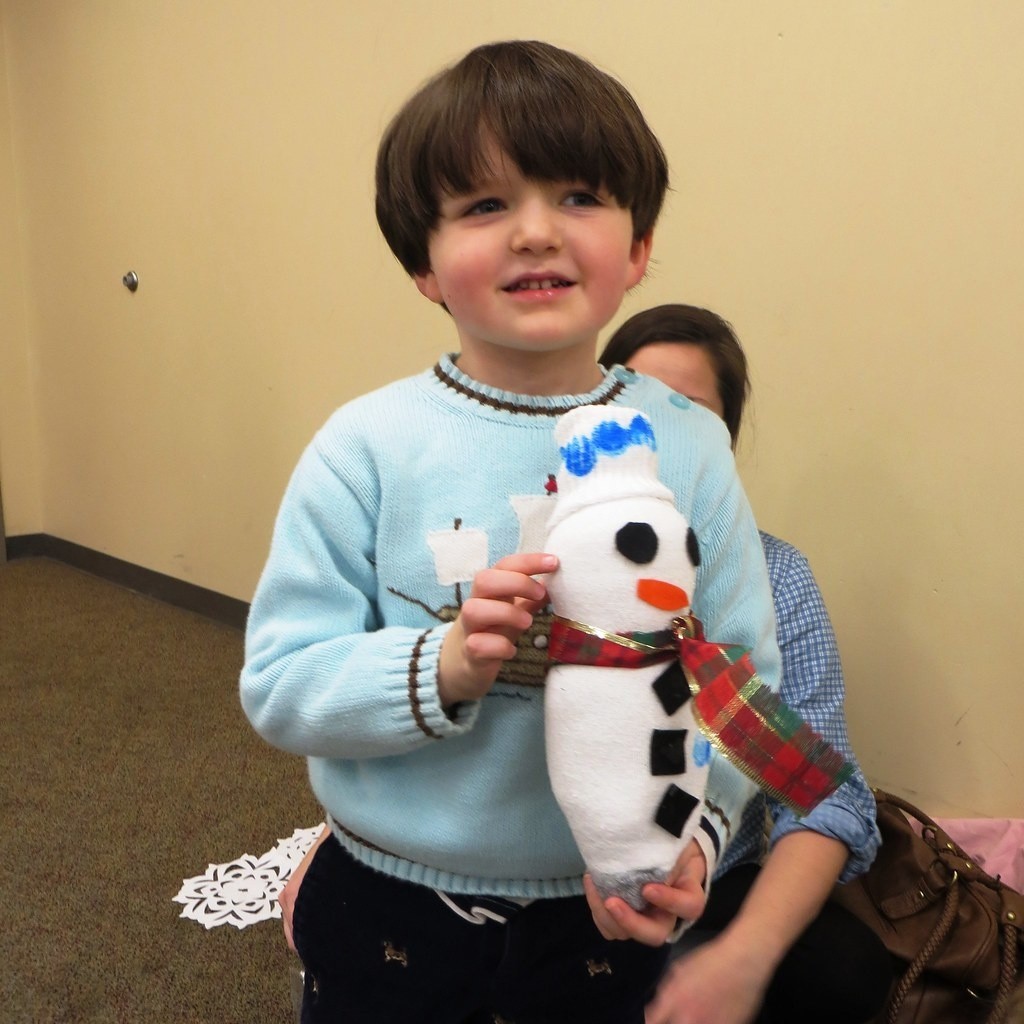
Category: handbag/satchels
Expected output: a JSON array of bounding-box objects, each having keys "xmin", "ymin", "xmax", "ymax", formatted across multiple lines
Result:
[{"xmin": 793, "ymin": 786, "xmax": 1024, "ymax": 1024}]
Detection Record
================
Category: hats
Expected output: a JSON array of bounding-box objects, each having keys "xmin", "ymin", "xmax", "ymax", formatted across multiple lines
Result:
[{"xmin": 544, "ymin": 402, "xmax": 673, "ymax": 538}]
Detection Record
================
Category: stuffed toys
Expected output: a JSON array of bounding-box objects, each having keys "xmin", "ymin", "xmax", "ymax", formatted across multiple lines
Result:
[{"xmin": 537, "ymin": 403, "xmax": 719, "ymax": 911}]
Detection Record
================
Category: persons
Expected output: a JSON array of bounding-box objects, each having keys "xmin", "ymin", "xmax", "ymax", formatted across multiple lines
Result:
[
  {"xmin": 281, "ymin": 302, "xmax": 883, "ymax": 1020},
  {"xmin": 237, "ymin": 51, "xmax": 790, "ymax": 1024}
]
